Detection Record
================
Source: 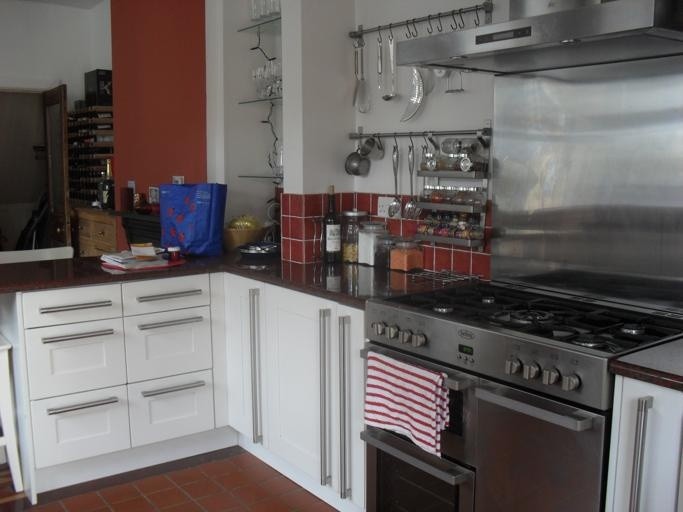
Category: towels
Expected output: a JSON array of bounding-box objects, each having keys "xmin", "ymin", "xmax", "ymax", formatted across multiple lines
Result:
[{"xmin": 363, "ymin": 352, "xmax": 452, "ymax": 459}]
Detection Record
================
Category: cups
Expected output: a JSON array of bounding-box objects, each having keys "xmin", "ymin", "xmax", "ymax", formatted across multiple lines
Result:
[
  {"xmin": 345, "ymin": 147, "xmax": 371, "ymax": 176},
  {"xmin": 249, "ymin": 0, "xmax": 280, "ymax": 21},
  {"xmin": 359, "ymin": 131, "xmax": 385, "ymax": 161},
  {"xmin": 267, "ymin": 144, "xmax": 283, "ymax": 177},
  {"xmin": 421, "ymin": 137, "xmax": 486, "ymax": 172}
]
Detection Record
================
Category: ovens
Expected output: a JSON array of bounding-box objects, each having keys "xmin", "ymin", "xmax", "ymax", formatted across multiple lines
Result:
[{"xmin": 359, "ymin": 340, "xmax": 608, "ymax": 512}]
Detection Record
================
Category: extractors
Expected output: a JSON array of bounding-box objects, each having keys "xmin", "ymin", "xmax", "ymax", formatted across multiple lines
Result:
[{"xmin": 396, "ymin": 0, "xmax": 682, "ymax": 76}]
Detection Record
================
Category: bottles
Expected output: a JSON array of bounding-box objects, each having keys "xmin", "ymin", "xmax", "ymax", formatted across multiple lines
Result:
[
  {"xmin": 66, "ymin": 110, "xmax": 116, "ymax": 211},
  {"xmin": 168, "ymin": 246, "xmax": 180, "ymax": 262},
  {"xmin": 419, "ymin": 211, "xmax": 484, "ymax": 240},
  {"xmin": 341, "ymin": 207, "xmax": 424, "ymax": 273},
  {"xmin": 321, "ymin": 260, "xmax": 423, "ymax": 301},
  {"xmin": 323, "ymin": 184, "xmax": 341, "ymax": 263},
  {"xmin": 420, "ymin": 185, "xmax": 485, "ymax": 207}
]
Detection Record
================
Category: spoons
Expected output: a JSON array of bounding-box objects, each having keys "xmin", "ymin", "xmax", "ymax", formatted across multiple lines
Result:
[
  {"xmin": 388, "ymin": 144, "xmax": 401, "ymax": 217},
  {"xmin": 402, "ymin": 144, "xmax": 417, "ymax": 218}
]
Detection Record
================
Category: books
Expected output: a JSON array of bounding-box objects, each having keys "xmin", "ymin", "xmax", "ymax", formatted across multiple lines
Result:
[{"xmin": 100, "ymin": 250, "xmax": 169, "ymax": 274}]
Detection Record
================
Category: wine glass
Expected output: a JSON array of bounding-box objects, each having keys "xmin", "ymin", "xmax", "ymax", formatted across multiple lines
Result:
[{"xmin": 251, "ymin": 60, "xmax": 282, "ymax": 98}]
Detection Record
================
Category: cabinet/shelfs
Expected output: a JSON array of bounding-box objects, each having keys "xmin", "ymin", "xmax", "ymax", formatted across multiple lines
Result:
[
  {"xmin": 599, "ymin": 375, "xmax": 680, "ymax": 512},
  {"xmin": 119, "ymin": 274, "xmax": 214, "ymax": 471},
  {"xmin": 71, "ymin": 208, "xmax": 127, "ymax": 257},
  {"xmin": 64, "ymin": 103, "xmax": 114, "ymax": 211},
  {"xmin": 283, "ymin": 285, "xmax": 365, "ymax": 511},
  {"xmin": 0, "ymin": 271, "xmax": 133, "ymax": 506},
  {"xmin": 229, "ymin": 0, "xmax": 283, "ymax": 180},
  {"xmin": 414, "ymin": 170, "xmax": 490, "ymax": 253},
  {"xmin": 211, "ymin": 273, "xmax": 283, "ymax": 471}
]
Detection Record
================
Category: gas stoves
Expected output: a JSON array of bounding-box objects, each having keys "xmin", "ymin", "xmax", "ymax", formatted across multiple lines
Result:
[{"xmin": 364, "ymin": 277, "xmax": 683, "ymax": 413}]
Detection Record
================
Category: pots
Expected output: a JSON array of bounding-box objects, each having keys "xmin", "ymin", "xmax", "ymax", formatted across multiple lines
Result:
[{"xmin": 239, "ymin": 242, "xmax": 280, "ymax": 261}]
